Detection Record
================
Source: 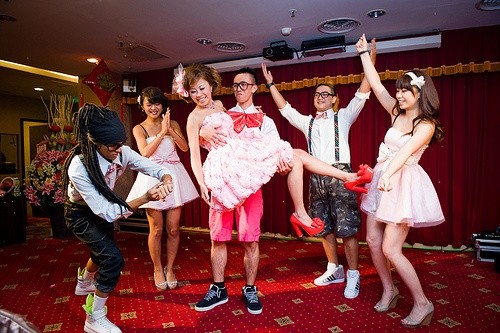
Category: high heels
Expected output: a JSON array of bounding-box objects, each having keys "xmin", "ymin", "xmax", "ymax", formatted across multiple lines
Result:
[
  {"xmin": 402, "ymin": 301, "xmax": 434, "ymax": 328},
  {"xmin": 375, "ymin": 286, "xmax": 399, "ymax": 312},
  {"xmin": 290, "ymin": 213, "xmax": 324, "ymax": 238},
  {"xmin": 343, "ymin": 163, "xmax": 374, "ymax": 194},
  {"xmin": 163, "ymin": 267, "xmax": 177, "ymax": 289},
  {"xmin": 153, "ymin": 271, "xmax": 166, "ymax": 291}
]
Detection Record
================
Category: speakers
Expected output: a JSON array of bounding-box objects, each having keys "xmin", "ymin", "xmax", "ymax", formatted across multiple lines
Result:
[{"xmin": 301, "ymin": 35, "xmax": 346, "ymax": 57}]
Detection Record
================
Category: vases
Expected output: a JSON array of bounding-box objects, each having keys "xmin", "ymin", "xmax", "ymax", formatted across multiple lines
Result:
[{"xmin": 48, "ymin": 207, "xmax": 65, "ymax": 239}]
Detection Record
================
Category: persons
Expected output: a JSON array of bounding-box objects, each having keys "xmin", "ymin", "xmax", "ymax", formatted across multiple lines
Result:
[
  {"xmin": 262, "ymin": 38, "xmax": 376, "ymax": 297},
  {"xmin": 65, "ymin": 102, "xmax": 173, "ymax": 333},
  {"xmin": 195, "ymin": 67, "xmax": 293, "ymax": 314},
  {"xmin": 125, "ymin": 87, "xmax": 200, "ymax": 290},
  {"xmin": 175, "ymin": 64, "xmax": 374, "ymax": 237},
  {"xmin": 356, "ymin": 34, "xmax": 446, "ymax": 326}
]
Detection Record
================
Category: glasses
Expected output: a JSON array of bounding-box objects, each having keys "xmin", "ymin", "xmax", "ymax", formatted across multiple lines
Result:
[
  {"xmin": 102, "ymin": 138, "xmax": 128, "ymax": 152},
  {"xmin": 313, "ymin": 92, "xmax": 333, "ymax": 98},
  {"xmin": 232, "ymin": 82, "xmax": 255, "ymax": 91}
]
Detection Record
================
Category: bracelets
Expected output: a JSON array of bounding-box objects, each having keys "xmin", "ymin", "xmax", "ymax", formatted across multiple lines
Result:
[
  {"xmin": 359, "ymin": 50, "xmax": 371, "ymax": 56},
  {"xmin": 266, "ymin": 82, "xmax": 274, "ymax": 89},
  {"xmin": 157, "ymin": 133, "xmax": 164, "ymax": 140}
]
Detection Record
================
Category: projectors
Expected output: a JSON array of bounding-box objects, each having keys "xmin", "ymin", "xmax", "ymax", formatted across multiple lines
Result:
[{"xmin": 263, "ymin": 40, "xmax": 293, "ymax": 62}]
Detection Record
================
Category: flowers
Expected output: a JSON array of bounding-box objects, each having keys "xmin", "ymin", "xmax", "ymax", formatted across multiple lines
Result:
[{"xmin": 23, "ymin": 93, "xmax": 78, "ymax": 208}]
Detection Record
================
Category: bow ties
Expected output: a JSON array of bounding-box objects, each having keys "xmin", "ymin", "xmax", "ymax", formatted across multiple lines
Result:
[
  {"xmin": 316, "ymin": 112, "xmax": 328, "ymax": 120},
  {"xmin": 226, "ymin": 111, "xmax": 262, "ymax": 132}
]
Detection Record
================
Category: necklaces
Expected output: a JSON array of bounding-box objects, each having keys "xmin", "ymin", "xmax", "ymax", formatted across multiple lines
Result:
[{"xmin": 201, "ymin": 101, "xmax": 217, "ymax": 110}]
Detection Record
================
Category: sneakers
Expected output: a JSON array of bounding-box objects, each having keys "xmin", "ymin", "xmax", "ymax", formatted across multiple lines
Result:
[
  {"xmin": 75, "ymin": 267, "xmax": 96, "ymax": 295},
  {"xmin": 344, "ymin": 270, "xmax": 360, "ymax": 299},
  {"xmin": 242, "ymin": 286, "xmax": 262, "ymax": 314},
  {"xmin": 82, "ymin": 293, "xmax": 122, "ymax": 333},
  {"xmin": 195, "ymin": 284, "xmax": 228, "ymax": 311},
  {"xmin": 314, "ymin": 262, "xmax": 344, "ymax": 286}
]
